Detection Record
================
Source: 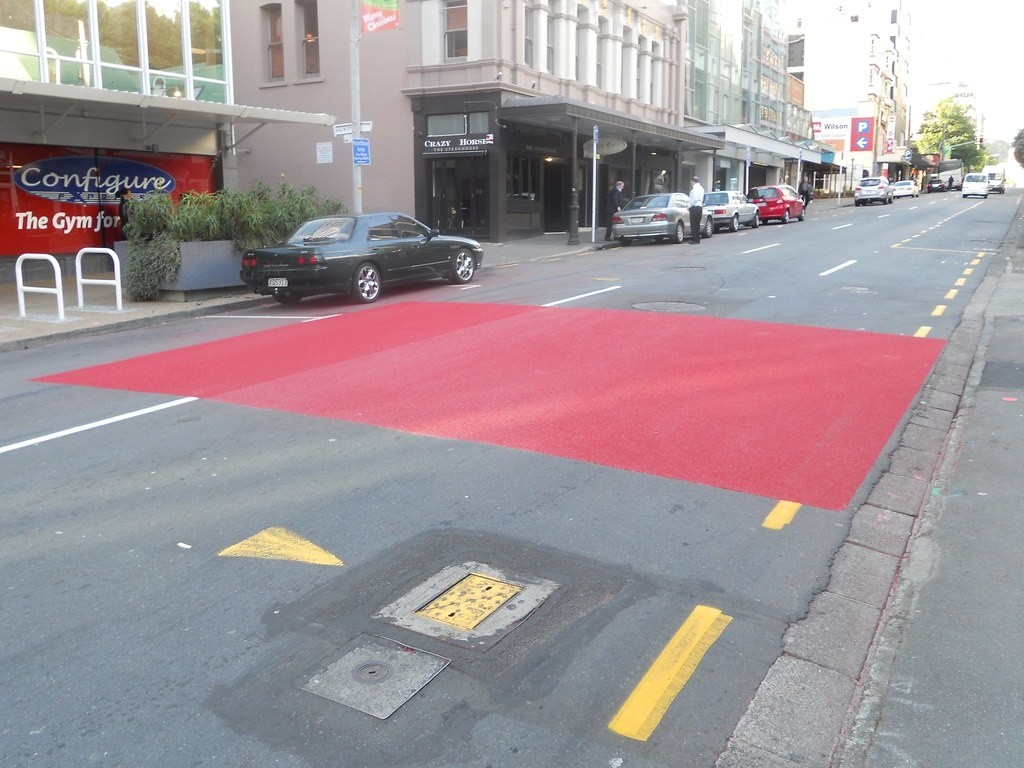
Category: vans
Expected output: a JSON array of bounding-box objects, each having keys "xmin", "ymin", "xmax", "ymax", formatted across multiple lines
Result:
[{"xmin": 981, "ymin": 165, "xmax": 1006, "ymax": 193}]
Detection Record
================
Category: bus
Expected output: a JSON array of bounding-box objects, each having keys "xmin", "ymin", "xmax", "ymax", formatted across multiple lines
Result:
[{"xmin": 936, "ymin": 159, "xmax": 965, "ymax": 190}]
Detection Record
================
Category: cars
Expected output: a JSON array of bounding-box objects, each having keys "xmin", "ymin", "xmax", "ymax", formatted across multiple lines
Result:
[
  {"xmin": 927, "ymin": 180, "xmax": 945, "ymax": 193},
  {"xmin": 892, "ymin": 179, "xmax": 920, "ymax": 199},
  {"xmin": 962, "ymin": 173, "xmax": 990, "ymax": 199},
  {"xmin": 240, "ymin": 210, "xmax": 484, "ymax": 305},
  {"xmin": 701, "ymin": 190, "xmax": 760, "ymax": 233},
  {"xmin": 612, "ymin": 192, "xmax": 714, "ymax": 244},
  {"xmin": 506, "ymin": 191, "xmax": 536, "ymax": 200},
  {"xmin": 744, "ymin": 184, "xmax": 806, "ymax": 225}
]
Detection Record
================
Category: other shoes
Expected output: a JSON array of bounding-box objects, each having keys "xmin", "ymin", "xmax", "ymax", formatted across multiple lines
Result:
[
  {"xmin": 688, "ymin": 240, "xmax": 700, "ymax": 244},
  {"xmin": 605, "ymin": 238, "xmax": 612, "ymax": 241}
]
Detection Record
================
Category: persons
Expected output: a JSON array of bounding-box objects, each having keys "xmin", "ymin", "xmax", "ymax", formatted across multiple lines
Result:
[
  {"xmin": 949, "ymin": 175, "xmax": 953, "ymax": 190},
  {"xmin": 798, "ymin": 176, "xmax": 812, "ymax": 210},
  {"xmin": 115, "ymin": 188, "xmax": 132, "ymax": 241},
  {"xmin": 910, "ymin": 174, "xmax": 913, "ymax": 180},
  {"xmin": 688, "ymin": 177, "xmax": 704, "ymax": 244},
  {"xmin": 605, "ymin": 180, "xmax": 624, "ymax": 241}
]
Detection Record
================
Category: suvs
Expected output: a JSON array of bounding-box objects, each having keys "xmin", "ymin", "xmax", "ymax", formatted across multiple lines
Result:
[{"xmin": 854, "ymin": 176, "xmax": 894, "ymax": 207}]
